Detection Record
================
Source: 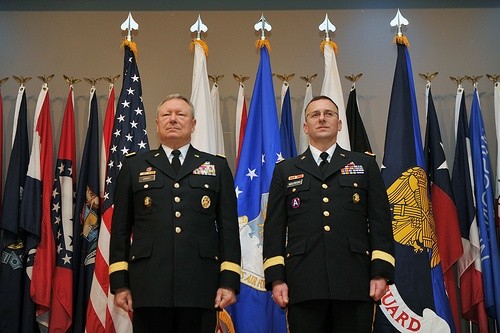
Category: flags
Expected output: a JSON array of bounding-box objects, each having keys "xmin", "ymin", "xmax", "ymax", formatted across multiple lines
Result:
[{"xmin": 0, "ymin": 38, "xmax": 500, "ymax": 333}]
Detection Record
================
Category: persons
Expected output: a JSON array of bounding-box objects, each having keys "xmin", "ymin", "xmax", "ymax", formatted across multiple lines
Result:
[
  {"xmin": 262, "ymin": 96, "xmax": 396, "ymax": 333},
  {"xmin": 108, "ymin": 94, "xmax": 241, "ymax": 333}
]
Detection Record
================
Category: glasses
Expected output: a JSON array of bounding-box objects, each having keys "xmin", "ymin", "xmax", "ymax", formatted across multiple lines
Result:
[{"xmin": 305, "ymin": 109, "xmax": 339, "ymax": 122}]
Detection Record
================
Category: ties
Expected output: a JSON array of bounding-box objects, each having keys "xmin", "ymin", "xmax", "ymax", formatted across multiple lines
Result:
[
  {"xmin": 318, "ymin": 152, "xmax": 330, "ymax": 171},
  {"xmin": 170, "ymin": 149, "xmax": 182, "ymax": 174}
]
